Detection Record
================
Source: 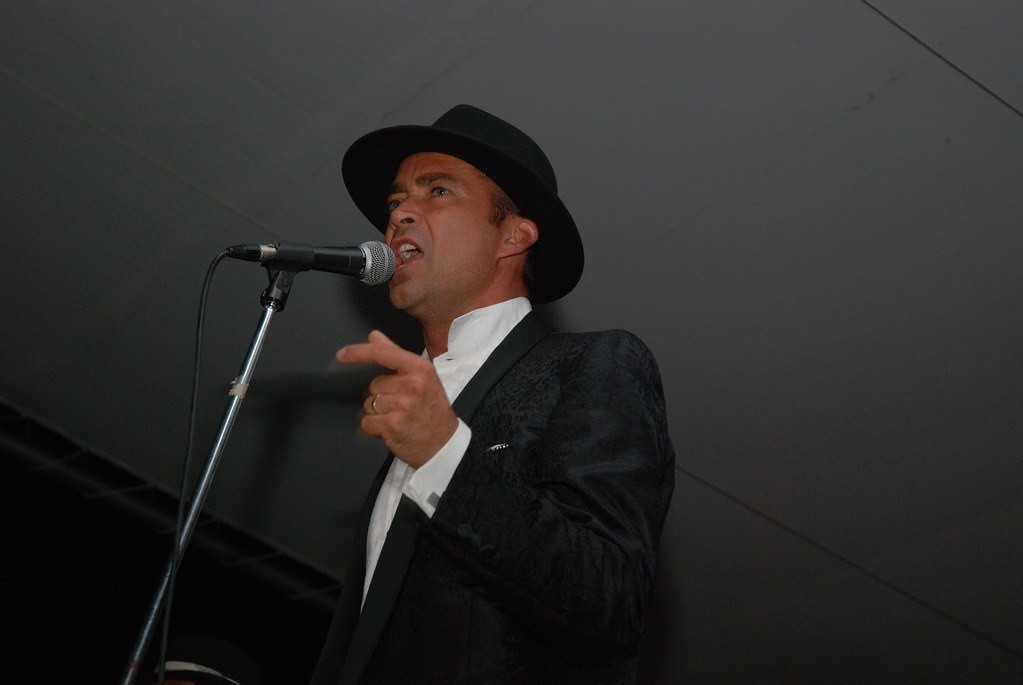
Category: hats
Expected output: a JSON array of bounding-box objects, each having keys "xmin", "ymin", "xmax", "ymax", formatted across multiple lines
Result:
[{"xmin": 341, "ymin": 104, "xmax": 585, "ymax": 304}]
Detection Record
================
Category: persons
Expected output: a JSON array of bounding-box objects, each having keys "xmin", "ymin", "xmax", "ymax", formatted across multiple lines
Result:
[
  {"xmin": 304, "ymin": 102, "xmax": 678, "ymax": 684},
  {"xmin": 152, "ymin": 630, "xmax": 261, "ymax": 685}
]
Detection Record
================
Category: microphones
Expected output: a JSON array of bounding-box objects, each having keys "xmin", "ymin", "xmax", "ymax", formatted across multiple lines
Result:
[{"xmin": 226, "ymin": 241, "xmax": 397, "ymax": 287}]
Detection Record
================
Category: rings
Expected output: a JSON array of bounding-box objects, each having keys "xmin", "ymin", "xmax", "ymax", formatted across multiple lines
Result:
[{"xmin": 371, "ymin": 393, "xmax": 381, "ymax": 413}]
